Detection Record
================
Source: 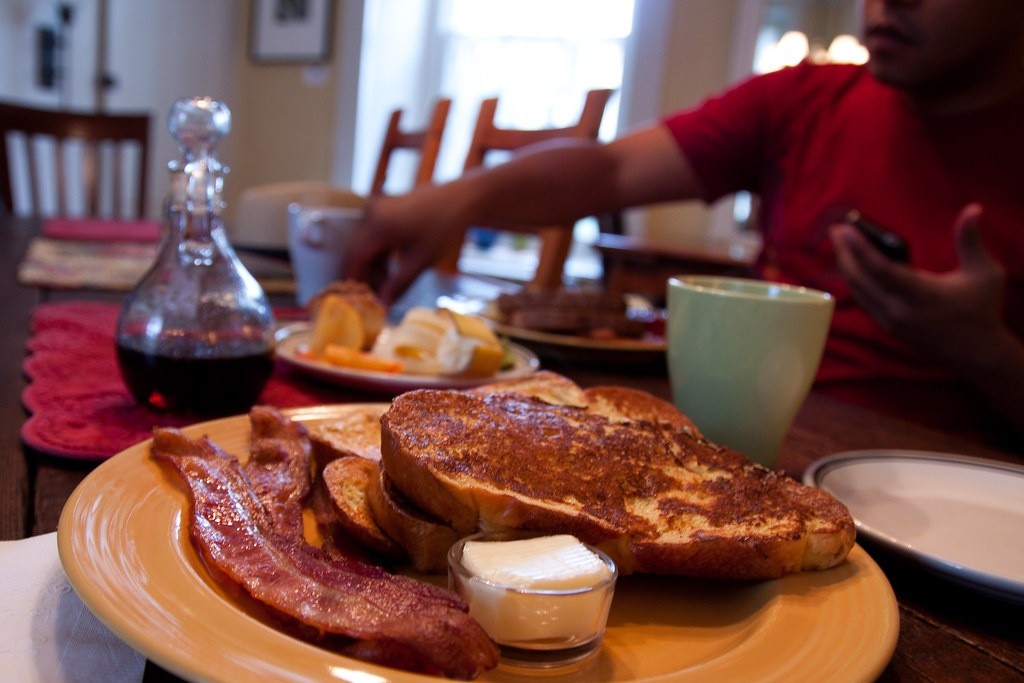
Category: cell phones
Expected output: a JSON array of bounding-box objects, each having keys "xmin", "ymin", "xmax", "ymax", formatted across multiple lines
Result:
[{"xmin": 846, "ymin": 208, "xmax": 911, "ymax": 263}]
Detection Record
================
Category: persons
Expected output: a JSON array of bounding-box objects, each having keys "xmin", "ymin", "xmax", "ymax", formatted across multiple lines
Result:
[{"xmin": 342, "ymin": 1, "xmax": 1023, "ymax": 446}]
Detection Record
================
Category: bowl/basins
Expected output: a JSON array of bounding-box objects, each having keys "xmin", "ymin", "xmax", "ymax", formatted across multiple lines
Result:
[{"xmin": 445, "ymin": 531, "xmax": 620, "ymax": 670}]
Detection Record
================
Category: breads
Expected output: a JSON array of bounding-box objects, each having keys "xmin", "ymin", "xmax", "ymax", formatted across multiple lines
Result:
[
  {"xmin": 308, "ymin": 372, "xmax": 857, "ymax": 577},
  {"xmin": 394, "ymin": 305, "xmax": 505, "ymax": 378},
  {"xmin": 308, "ymin": 294, "xmax": 383, "ymax": 352}
]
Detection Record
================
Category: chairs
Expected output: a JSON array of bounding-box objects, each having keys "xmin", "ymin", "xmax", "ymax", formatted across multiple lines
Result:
[
  {"xmin": 0, "ymin": 98, "xmax": 154, "ymax": 217},
  {"xmin": 371, "ymin": 96, "xmax": 452, "ymax": 198},
  {"xmin": 460, "ymin": 88, "xmax": 614, "ymax": 243}
]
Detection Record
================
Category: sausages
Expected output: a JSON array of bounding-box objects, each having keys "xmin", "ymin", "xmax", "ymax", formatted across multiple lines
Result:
[{"xmin": 497, "ymin": 291, "xmax": 649, "ymax": 340}]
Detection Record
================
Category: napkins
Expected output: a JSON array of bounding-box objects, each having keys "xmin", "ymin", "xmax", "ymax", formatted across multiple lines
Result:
[{"xmin": 0, "ymin": 531, "xmax": 147, "ymax": 683}]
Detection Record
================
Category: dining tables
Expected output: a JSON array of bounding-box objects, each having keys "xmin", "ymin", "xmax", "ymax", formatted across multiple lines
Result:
[{"xmin": 0, "ymin": 213, "xmax": 1024, "ymax": 683}]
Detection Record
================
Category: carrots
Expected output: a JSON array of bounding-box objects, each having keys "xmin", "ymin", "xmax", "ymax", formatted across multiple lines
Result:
[{"xmin": 321, "ymin": 343, "xmax": 402, "ymax": 372}]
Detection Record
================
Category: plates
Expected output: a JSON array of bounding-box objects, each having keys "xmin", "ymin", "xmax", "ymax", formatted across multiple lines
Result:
[
  {"xmin": 272, "ymin": 320, "xmax": 540, "ymax": 389},
  {"xmin": 56, "ymin": 401, "xmax": 901, "ymax": 683},
  {"xmin": 805, "ymin": 449, "xmax": 1024, "ymax": 591},
  {"xmin": 434, "ymin": 291, "xmax": 666, "ymax": 349}
]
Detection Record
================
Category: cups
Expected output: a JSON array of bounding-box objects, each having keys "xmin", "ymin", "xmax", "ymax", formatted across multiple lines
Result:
[
  {"xmin": 667, "ymin": 274, "xmax": 835, "ymax": 461},
  {"xmin": 288, "ymin": 203, "xmax": 364, "ymax": 309}
]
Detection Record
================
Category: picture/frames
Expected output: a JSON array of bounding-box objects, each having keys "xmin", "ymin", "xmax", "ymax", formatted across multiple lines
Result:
[{"xmin": 246, "ymin": 0, "xmax": 337, "ymax": 66}]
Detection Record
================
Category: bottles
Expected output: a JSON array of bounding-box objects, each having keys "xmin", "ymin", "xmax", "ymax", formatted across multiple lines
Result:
[{"xmin": 114, "ymin": 95, "xmax": 271, "ymax": 418}]
